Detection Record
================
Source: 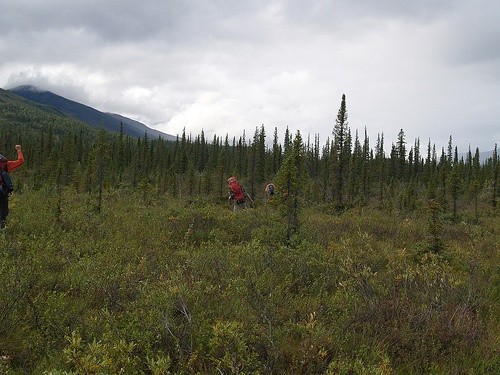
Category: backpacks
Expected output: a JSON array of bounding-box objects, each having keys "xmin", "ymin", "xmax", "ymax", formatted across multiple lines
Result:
[{"xmin": 0, "ymin": 165, "xmax": 14, "ymax": 197}]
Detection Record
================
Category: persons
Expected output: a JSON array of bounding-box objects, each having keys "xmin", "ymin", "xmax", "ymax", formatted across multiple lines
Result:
[
  {"xmin": 0, "ymin": 145, "xmax": 26, "ymax": 231},
  {"xmin": 266, "ymin": 182, "xmax": 279, "ymax": 208},
  {"xmin": 226, "ymin": 176, "xmax": 254, "ymax": 212}
]
